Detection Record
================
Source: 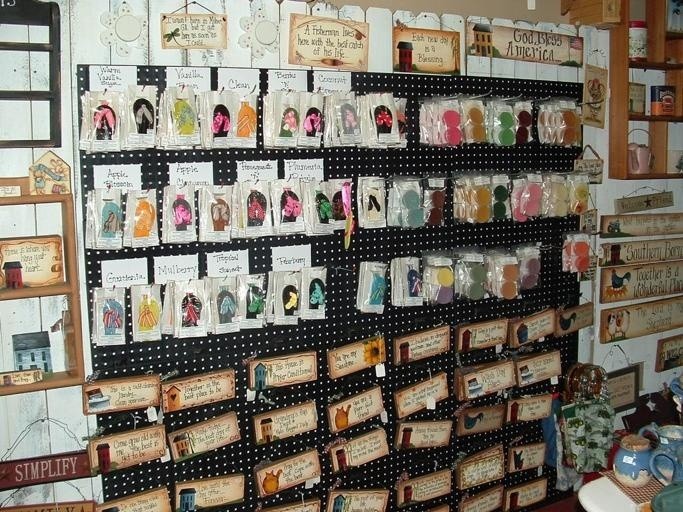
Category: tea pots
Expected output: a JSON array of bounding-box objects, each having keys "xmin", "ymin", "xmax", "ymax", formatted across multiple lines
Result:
[{"xmin": 639, "ymin": 421, "xmax": 683, "ymax": 488}]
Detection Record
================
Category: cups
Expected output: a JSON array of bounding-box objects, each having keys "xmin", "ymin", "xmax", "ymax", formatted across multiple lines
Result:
[{"xmin": 629, "ymin": 20, "xmax": 647, "ymax": 63}]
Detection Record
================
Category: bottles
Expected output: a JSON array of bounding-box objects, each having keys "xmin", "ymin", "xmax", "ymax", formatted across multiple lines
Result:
[{"xmin": 613, "ymin": 433, "xmax": 654, "ymax": 490}]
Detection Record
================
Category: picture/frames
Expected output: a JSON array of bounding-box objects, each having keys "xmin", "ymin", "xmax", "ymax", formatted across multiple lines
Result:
[{"xmin": 605, "ymin": 363, "xmax": 640, "ymax": 414}]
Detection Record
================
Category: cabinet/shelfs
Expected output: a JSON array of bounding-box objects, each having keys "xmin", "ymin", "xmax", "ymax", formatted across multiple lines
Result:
[
  {"xmin": 608, "ymin": 0, "xmax": 683, "ymax": 180},
  {"xmin": 0, "ymin": 0, "xmax": 61, "ymax": 149},
  {"xmin": 0, "ymin": 177, "xmax": 87, "ymax": 397}
]
